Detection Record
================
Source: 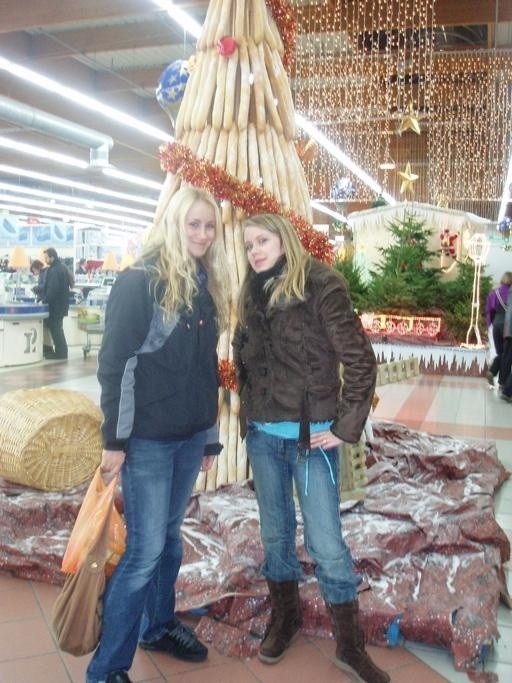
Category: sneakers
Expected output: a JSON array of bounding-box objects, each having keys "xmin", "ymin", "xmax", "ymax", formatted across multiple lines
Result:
[
  {"xmin": 107, "ymin": 671, "xmax": 130, "ymax": 683},
  {"xmin": 138, "ymin": 622, "xmax": 207, "ymax": 662}
]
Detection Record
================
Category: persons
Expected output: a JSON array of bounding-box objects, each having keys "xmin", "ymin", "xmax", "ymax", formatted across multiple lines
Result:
[
  {"xmin": 87, "ymin": 186, "xmax": 222, "ymax": 682},
  {"xmin": 232, "ymin": 213, "xmax": 392, "ymax": 682},
  {"xmin": 30, "ymin": 259, "xmax": 54, "ymax": 356},
  {"xmin": 42, "ymin": 248, "xmax": 74, "ymax": 358},
  {"xmin": 486, "ymin": 271, "xmax": 512, "ymax": 385},
  {"xmin": 497, "ymin": 286, "xmax": 511, "ymax": 403},
  {"xmin": 74, "ymin": 257, "xmax": 87, "ymax": 273}
]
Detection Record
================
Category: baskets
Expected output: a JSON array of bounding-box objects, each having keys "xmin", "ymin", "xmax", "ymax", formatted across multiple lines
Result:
[{"xmin": 0, "ymin": 388, "xmax": 105, "ymax": 491}]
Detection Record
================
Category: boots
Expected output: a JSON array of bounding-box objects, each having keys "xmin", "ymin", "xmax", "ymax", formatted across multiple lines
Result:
[
  {"xmin": 258, "ymin": 578, "xmax": 301, "ymax": 662},
  {"xmin": 326, "ymin": 601, "xmax": 390, "ymax": 683}
]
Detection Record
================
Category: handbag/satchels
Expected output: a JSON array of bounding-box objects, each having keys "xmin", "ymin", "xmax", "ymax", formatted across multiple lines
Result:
[{"xmin": 51, "ymin": 549, "xmax": 106, "ymax": 656}]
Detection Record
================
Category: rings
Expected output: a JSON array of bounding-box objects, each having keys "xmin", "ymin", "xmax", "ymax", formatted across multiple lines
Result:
[{"xmin": 323, "ymin": 438, "xmax": 328, "ymax": 444}]
